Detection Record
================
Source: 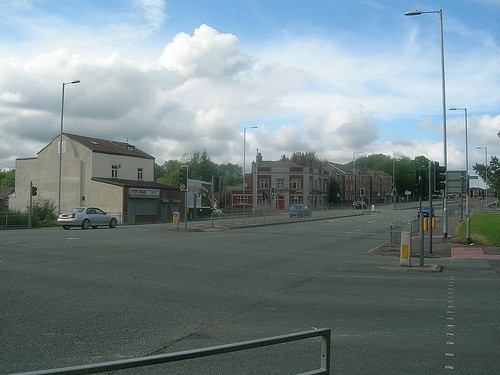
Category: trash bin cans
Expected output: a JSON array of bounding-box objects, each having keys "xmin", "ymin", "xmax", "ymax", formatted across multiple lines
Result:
[
  {"xmin": 430, "ymin": 211, "xmax": 436, "ymax": 228},
  {"xmin": 418, "ymin": 211, "xmax": 428, "ymax": 231}
]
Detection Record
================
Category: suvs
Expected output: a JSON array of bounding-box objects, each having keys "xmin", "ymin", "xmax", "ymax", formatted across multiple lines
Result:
[
  {"xmin": 289, "ymin": 204, "xmax": 312, "ymax": 218},
  {"xmin": 353, "ymin": 201, "xmax": 368, "ymax": 210}
]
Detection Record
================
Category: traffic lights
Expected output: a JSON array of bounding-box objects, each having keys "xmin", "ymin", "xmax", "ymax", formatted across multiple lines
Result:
[
  {"xmin": 415, "ymin": 171, "xmax": 426, "ymax": 196},
  {"xmin": 31, "ymin": 186, "xmax": 38, "ymax": 197},
  {"xmin": 360, "ymin": 188, "xmax": 366, "ymax": 197},
  {"xmin": 392, "ymin": 189, "xmax": 398, "ymax": 196},
  {"xmin": 434, "ymin": 161, "xmax": 446, "ymax": 195},
  {"xmin": 213, "ymin": 177, "xmax": 221, "ymax": 193},
  {"xmin": 179, "ymin": 165, "xmax": 188, "ymax": 191}
]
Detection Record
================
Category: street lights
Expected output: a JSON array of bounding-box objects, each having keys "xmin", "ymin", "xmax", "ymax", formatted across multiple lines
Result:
[
  {"xmin": 242, "ymin": 126, "xmax": 258, "ymax": 217},
  {"xmin": 404, "ymin": 8, "xmax": 449, "ymax": 239},
  {"xmin": 448, "ymin": 108, "xmax": 469, "ymax": 216},
  {"xmin": 393, "ymin": 159, "xmax": 401, "ymax": 188},
  {"xmin": 353, "ymin": 151, "xmax": 363, "ymax": 203},
  {"xmin": 58, "ymin": 79, "xmax": 81, "ymax": 214},
  {"xmin": 475, "ymin": 146, "xmax": 488, "ymax": 207}
]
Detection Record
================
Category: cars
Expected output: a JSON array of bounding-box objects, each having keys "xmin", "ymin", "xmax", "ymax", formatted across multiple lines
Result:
[
  {"xmin": 56, "ymin": 207, "xmax": 118, "ymax": 230},
  {"xmin": 417, "ymin": 205, "xmax": 435, "ymax": 218}
]
